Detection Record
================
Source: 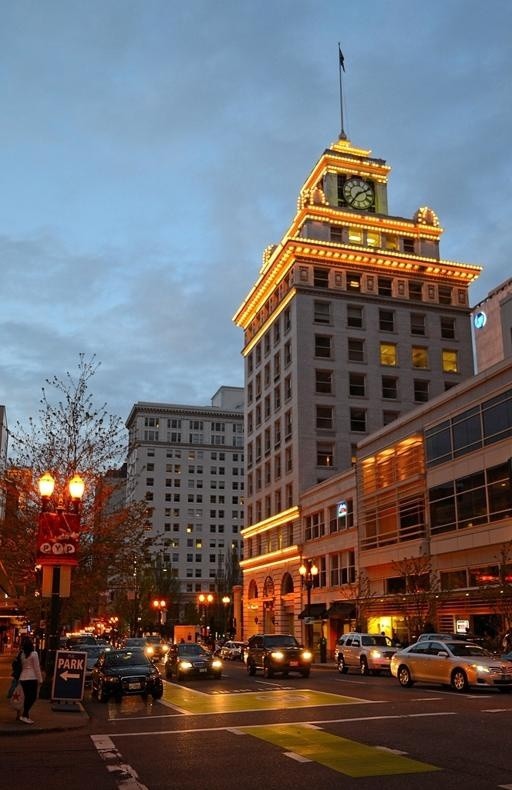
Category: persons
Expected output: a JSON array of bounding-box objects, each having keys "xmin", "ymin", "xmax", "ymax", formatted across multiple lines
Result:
[
  {"xmin": 483, "ymin": 631, "xmax": 492, "ymax": 643},
  {"xmin": 6, "ymin": 635, "xmax": 31, "ymax": 700},
  {"xmin": 391, "ymin": 632, "xmax": 400, "ymax": 647},
  {"xmin": 18, "ymin": 638, "xmax": 43, "ymax": 724},
  {"xmin": 378, "ymin": 632, "xmax": 387, "ymax": 645},
  {"xmin": 465, "ymin": 627, "xmax": 472, "ymax": 634}
]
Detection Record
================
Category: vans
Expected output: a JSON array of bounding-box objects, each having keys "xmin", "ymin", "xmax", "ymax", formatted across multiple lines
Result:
[
  {"xmin": 417, "ymin": 633, "xmax": 467, "ymax": 644},
  {"xmin": 125, "ymin": 637, "xmax": 168, "ymax": 660}
]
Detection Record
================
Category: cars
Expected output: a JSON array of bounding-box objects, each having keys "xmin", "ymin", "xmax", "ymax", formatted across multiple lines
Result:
[
  {"xmin": 165, "ymin": 643, "xmax": 222, "ymax": 681},
  {"xmin": 78, "ymin": 642, "xmax": 106, "ymax": 687},
  {"xmin": 92, "ymin": 647, "xmax": 164, "ymax": 703},
  {"xmin": 390, "ymin": 641, "xmax": 512, "ymax": 689},
  {"xmin": 38, "ymin": 633, "xmax": 96, "ymax": 651}
]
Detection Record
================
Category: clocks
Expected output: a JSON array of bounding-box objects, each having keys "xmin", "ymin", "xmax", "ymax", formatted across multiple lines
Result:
[{"xmin": 342, "ymin": 177, "xmax": 374, "ymax": 210}]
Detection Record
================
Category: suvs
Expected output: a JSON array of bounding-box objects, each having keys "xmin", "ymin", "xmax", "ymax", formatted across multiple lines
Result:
[
  {"xmin": 243, "ymin": 634, "xmax": 311, "ymax": 680},
  {"xmin": 334, "ymin": 632, "xmax": 403, "ymax": 674},
  {"xmin": 221, "ymin": 640, "xmax": 243, "ymax": 661}
]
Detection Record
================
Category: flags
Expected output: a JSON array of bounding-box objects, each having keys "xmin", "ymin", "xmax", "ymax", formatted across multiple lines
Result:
[{"xmin": 338, "ymin": 47, "xmax": 346, "ymax": 73}]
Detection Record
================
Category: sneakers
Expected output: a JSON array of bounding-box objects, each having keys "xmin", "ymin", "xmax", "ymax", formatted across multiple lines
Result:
[{"xmin": 20, "ymin": 715, "xmax": 35, "ymax": 724}]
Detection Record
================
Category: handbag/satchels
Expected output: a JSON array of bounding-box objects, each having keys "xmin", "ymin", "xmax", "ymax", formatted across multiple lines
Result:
[{"xmin": 8, "ymin": 684, "xmax": 26, "ymax": 712}]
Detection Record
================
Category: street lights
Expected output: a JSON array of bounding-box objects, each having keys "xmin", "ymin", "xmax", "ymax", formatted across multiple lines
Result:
[
  {"xmin": 109, "ymin": 617, "xmax": 119, "ymax": 648},
  {"xmin": 199, "ymin": 593, "xmax": 230, "ymax": 652},
  {"xmin": 36, "ymin": 472, "xmax": 87, "ymax": 696},
  {"xmin": 154, "ymin": 598, "xmax": 166, "ymax": 635},
  {"xmin": 299, "ymin": 558, "xmax": 318, "ymax": 647}
]
